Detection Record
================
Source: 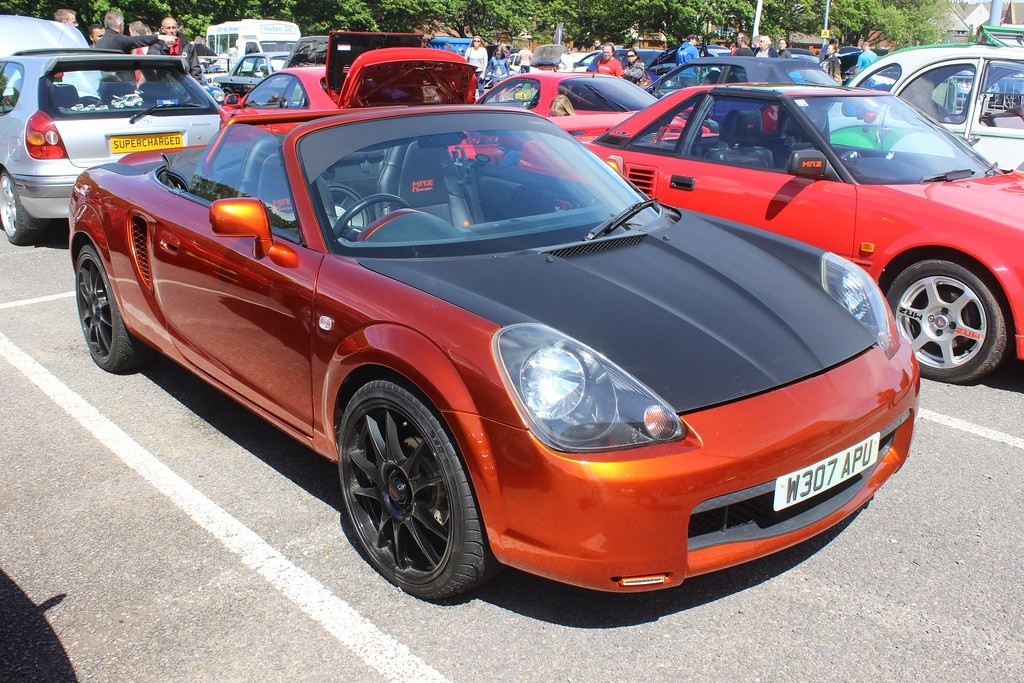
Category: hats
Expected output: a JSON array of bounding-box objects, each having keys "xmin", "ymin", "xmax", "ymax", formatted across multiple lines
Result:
[
  {"xmin": 687, "ymin": 34, "xmax": 700, "ymax": 40},
  {"xmin": 195, "ymin": 36, "xmax": 202, "ymax": 44}
]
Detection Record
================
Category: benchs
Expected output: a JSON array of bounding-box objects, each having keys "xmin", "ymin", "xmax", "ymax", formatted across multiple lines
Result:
[{"xmin": 54, "ymin": 83, "xmax": 170, "ymax": 107}]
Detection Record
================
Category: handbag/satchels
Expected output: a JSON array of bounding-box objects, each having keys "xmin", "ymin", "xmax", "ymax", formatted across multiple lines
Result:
[{"xmin": 635, "ymin": 69, "xmax": 653, "ymax": 91}]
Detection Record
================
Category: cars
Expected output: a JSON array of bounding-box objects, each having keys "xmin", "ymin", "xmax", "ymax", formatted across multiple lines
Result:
[
  {"xmin": 644, "ymin": 57, "xmax": 840, "ymax": 126},
  {"xmin": 217, "ymin": 31, "xmax": 504, "ymax": 168},
  {"xmin": 563, "ymin": 46, "xmax": 863, "ymax": 72},
  {"xmin": 65, "ymin": 99, "xmax": 921, "ymax": 603},
  {"xmin": 823, "ymin": 43, "xmax": 1024, "ymax": 174},
  {"xmin": 212, "ymin": 35, "xmax": 329, "ymax": 103},
  {"xmin": 519, "ymin": 83, "xmax": 1024, "ymax": 384},
  {"xmin": 484, "ymin": 44, "xmax": 564, "ymax": 88},
  {"xmin": 0, "ymin": 14, "xmax": 221, "ymax": 246},
  {"xmin": 476, "ymin": 72, "xmax": 709, "ymax": 139}
]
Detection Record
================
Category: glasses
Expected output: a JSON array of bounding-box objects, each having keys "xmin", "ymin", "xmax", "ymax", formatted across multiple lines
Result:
[
  {"xmin": 758, "ymin": 40, "xmax": 764, "ymax": 43},
  {"xmin": 473, "ymin": 40, "xmax": 481, "ymax": 43},
  {"xmin": 495, "ymin": 52, "xmax": 503, "ymax": 56},
  {"xmin": 97, "ymin": 90, "xmax": 100, "ymax": 93},
  {"xmin": 627, "ymin": 54, "xmax": 636, "ymax": 57}
]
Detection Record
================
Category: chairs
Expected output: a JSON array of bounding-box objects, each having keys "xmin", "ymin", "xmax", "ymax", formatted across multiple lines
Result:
[
  {"xmin": 376, "ymin": 140, "xmax": 474, "ymax": 227},
  {"xmin": 101, "ymin": 83, "xmax": 136, "ymax": 104},
  {"xmin": 989, "ymin": 113, "xmax": 1024, "ymax": 129},
  {"xmin": 239, "ymin": 134, "xmax": 338, "ymax": 228},
  {"xmin": 705, "ymin": 110, "xmax": 775, "ymax": 170},
  {"xmin": 784, "ymin": 110, "xmax": 826, "ymax": 152}
]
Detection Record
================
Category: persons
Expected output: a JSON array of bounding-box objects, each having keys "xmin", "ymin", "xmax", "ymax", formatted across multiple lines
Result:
[
  {"xmin": 817, "ymin": 39, "xmax": 843, "ymax": 86},
  {"xmin": 675, "ymin": 34, "xmax": 721, "ymax": 86},
  {"xmin": 463, "ymin": 35, "xmax": 533, "ymax": 98},
  {"xmin": 546, "ymin": 95, "xmax": 576, "ymax": 117},
  {"xmin": 53, "ymin": 9, "xmax": 219, "ymax": 80},
  {"xmin": 597, "ymin": 42, "xmax": 645, "ymax": 88},
  {"xmin": 855, "ymin": 41, "xmax": 879, "ymax": 76},
  {"xmin": 730, "ymin": 31, "xmax": 792, "ymax": 58}
]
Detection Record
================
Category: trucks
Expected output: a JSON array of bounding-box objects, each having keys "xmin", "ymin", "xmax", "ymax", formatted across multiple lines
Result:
[{"xmin": 206, "ymin": 17, "xmax": 301, "ymax": 71}]
